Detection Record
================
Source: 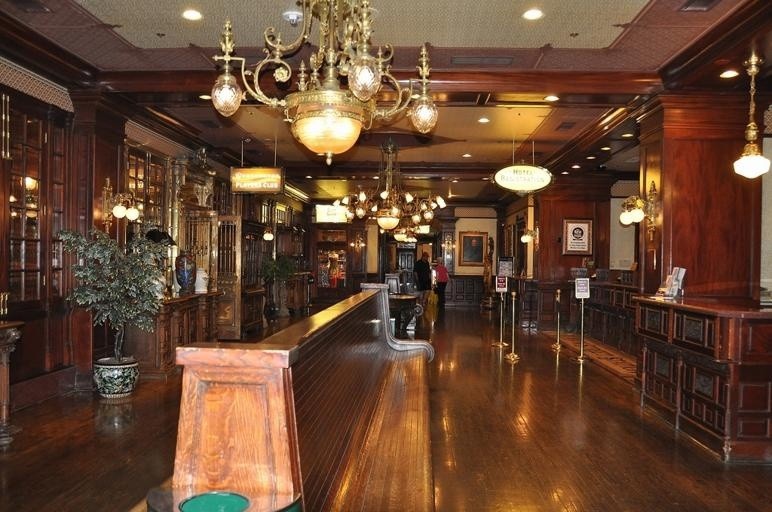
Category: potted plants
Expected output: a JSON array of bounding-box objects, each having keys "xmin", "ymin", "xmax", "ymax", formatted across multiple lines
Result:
[{"xmin": 50, "ymin": 227, "xmax": 175, "ymax": 400}]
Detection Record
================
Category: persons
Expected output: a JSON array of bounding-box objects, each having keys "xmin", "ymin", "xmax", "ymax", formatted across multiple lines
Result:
[
  {"xmin": 464, "ymin": 238, "xmax": 481, "ymax": 261},
  {"xmin": 435, "ymin": 256, "xmax": 452, "ymax": 311},
  {"xmin": 482, "ymin": 254, "xmax": 493, "ymax": 294},
  {"xmin": 412, "ymin": 251, "xmax": 432, "ymax": 308},
  {"xmin": 433, "ymin": 311, "xmax": 450, "ymax": 338}
]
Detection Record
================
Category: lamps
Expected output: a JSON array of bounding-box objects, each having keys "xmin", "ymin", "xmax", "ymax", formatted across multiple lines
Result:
[
  {"xmin": 732, "ymin": 39, "xmax": 771, "ymax": 181},
  {"xmin": 618, "ymin": 180, "xmax": 661, "ymax": 241},
  {"xmin": 331, "ymin": 137, "xmax": 447, "ymax": 232},
  {"xmin": 99, "ymin": 177, "xmax": 141, "ymax": 229},
  {"xmin": 211, "ymin": 2, "xmax": 439, "ymax": 166}
]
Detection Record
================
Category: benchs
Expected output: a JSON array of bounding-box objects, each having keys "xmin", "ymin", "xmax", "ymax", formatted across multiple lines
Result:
[{"xmin": 128, "ymin": 283, "xmax": 436, "ymax": 511}]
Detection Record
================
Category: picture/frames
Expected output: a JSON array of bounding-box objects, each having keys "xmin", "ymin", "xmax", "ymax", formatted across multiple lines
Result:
[
  {"xmin": 562, "ymin": 216, "xmax": 595, "ymax": 257},
  {"xmin": 457, "ymin": 230, "xmax": 489, "ymax": 268}
]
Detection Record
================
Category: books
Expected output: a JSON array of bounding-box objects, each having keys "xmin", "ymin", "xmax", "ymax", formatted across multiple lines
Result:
[
  {"xmin": 760, "ymin": 291, "xmax": 772, "ymax": 302},
  {"xmin": 760, "ymin": 302, "xmax": 772, "ymax": 306},
  {"xmin": 677, "ymin": 266, "xmax": 689, "ymax": 292},
  {"xmin": 671, "ymin": 266, "xmax": 680, "ymax": 282},
  {"xmin": 665, "ymin": 275, "xmax": 675, "ymax": 287},
  {"xmin": 655, "ymin": 280, "xmax": 678, "ymax": 300}
]
